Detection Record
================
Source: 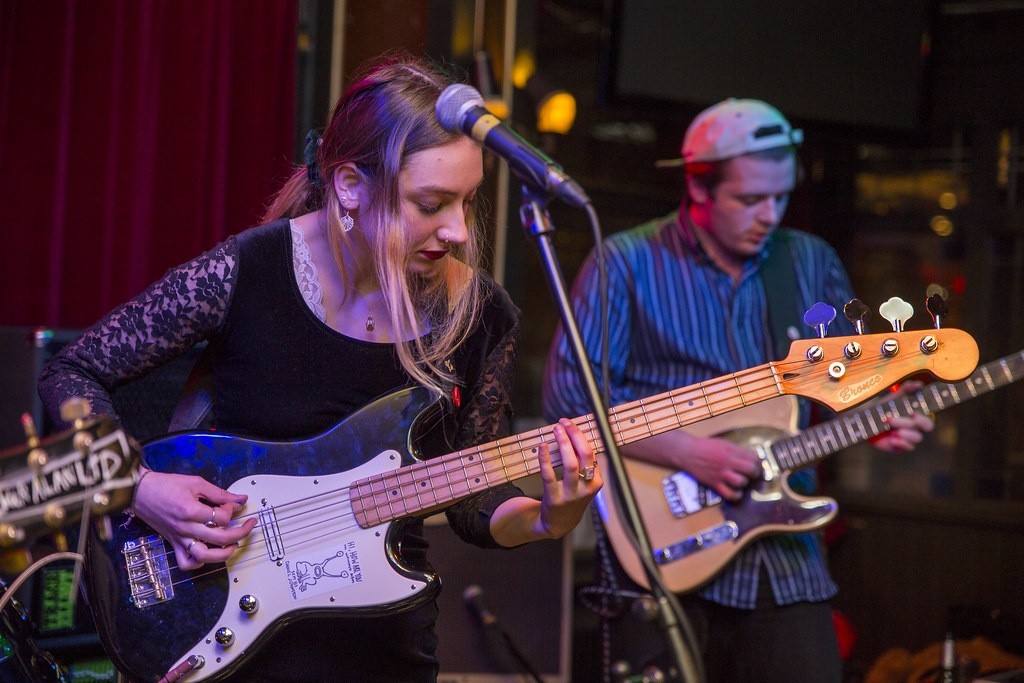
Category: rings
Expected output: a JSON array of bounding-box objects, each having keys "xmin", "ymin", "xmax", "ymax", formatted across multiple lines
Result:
[
  {"xmin": 579, "ymin": 465, "xmax": 595, "ymax": 480},
  {"xmin": 185, "ymin": 539, "xmax": 198, "ymax": 556},
  {"xmin": 205, "ymin": 508, "xmax": 216, "ymax": 528},
  {"xmin": 593, "ymin": 460, "xmax": 598, "ymax": 467}
]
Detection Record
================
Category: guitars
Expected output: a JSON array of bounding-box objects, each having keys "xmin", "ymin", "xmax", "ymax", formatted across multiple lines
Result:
[
  {"xmin": 594, "ymin": 329, "xmax": 1024, "ymax": 592},
  {"xmin": 1, "ymin": 393, "xmax": 140, "ymax": 570},
  {"xmin": 90, "ymin": 292, "xmax": 979, "ymax": 683}
]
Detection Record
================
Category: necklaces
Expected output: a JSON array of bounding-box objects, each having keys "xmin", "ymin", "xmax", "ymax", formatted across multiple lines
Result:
[{"xmin": 317, "ymin": 210, "xmax": 385, "ymax": 333}]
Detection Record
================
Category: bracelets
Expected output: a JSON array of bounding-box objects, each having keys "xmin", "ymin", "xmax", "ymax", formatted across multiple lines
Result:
[{"xmin": 118, "ymin": 469, "xmax": 152, "ymax": 531}]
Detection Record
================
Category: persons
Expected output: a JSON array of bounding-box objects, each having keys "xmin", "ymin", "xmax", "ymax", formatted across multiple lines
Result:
[
  {"xmin": 39, "ymin": 55, "xmax": 603, "ymax": 681},
  {"xmin": 544, "ymin": 95, "xmax": 936, "ymax": 682}
]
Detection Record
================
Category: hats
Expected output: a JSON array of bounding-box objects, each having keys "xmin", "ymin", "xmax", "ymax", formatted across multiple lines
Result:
[{"xmin": 656, "ymin": 97, "xmax": 803, "ymax": 167}]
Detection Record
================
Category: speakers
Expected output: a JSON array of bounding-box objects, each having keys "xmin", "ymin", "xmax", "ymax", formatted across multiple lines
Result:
[
  {"xmin": 420, "ymin": 508, "xmax": 576, "ymax": 683},
  {"xmin": 823, "ymin": 486, "xmax": 1024, "ymax": 683}
]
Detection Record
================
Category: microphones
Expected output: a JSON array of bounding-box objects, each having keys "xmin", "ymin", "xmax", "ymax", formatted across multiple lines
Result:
[
  {"xmin": 434, "ymin": 84, "xmax": 591, "ymax": 209},
  {"xmin": 463, "ymin": 584, "xmax": 494, "ymax": 626}
]
